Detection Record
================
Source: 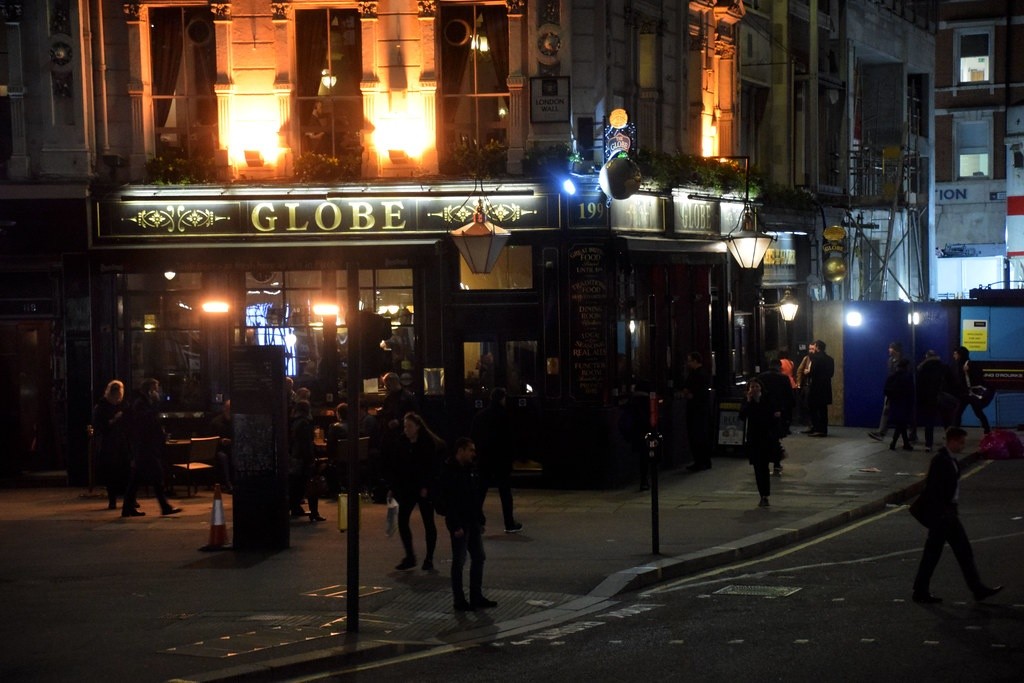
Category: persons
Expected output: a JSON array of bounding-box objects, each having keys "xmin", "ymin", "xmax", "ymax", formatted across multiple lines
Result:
[
  {"xmin": 908, "ymin": 426, "xmax": 1004, "ymax": 605},
  {"xmin": 91, "ymin": 380, "xmax": 140, "ymax": 510},
  {"xmin": 476, "ymin": 352, "xmax": 526, "ymax": 393},
  {"xmin": 286, "ymin": 372, "xmax": 376, "ymax": 525},
  {"xmin": 883, "ymin": 359, "xmax": 913, "ymax": 451},
  {"xmin": 738, "ymin": 376, "xmax": 782, "ymax": 506},
  {"xmin": 620, "ymin": 399, "xmax": 651, "ymax": 491},
  {"xmin": 752, "ymin": 340, "xmax": 834, "ymax": 477},
  {"xmin": 371, "ymin": 372, "xmax": 417, "ymax": 504},
  {"xmin": 211, "ymin": 400, "xmax": 235, "ymax": 494},
  {"xmin": 122, "ymin": 378, "xmax": 181, "ymax": 517},
  {"xmin": 867, "ymin": 341, "xmax": 918, "ymax": 442},
  {"xmin": 387, "ymin": 412, "xmax": 446, "ymax": 570},
  {"xmin": 680, "ymin": 352, "xmax": 712, "ymax": 472},
  {"xmin": 917, "ymin": 349, "xmax": 952, "ymax": 453},
  {"xmin": 478, "ymin": 387, "xmax": 522, "ymax": 533},
  {"xmin": 943, "ymin": 347, "xmax": 992, "ymax": 439},
  {"xmin": 432, "ymin": 436, "xmax": 497, "ymax": 611}
]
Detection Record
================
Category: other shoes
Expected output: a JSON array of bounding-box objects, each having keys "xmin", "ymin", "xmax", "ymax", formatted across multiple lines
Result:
[
  {"xmin": 800, "ymin": 428, "xmax": 933, "ymax": 452},
  {"xmin": 395, "ymin": 559, "xmax": 417, "ymax": 570},
  {"xmin": 223, "ymin": 486, "xmax": 233, "ymax": 492},
  {"xmin": 773, "ymin": 465, "xmax": 783, "ymax": 476},
  {"xmin": 162, "ymin": 508, "xmax": 183, "ymax": 515},
  {"xmin": 470, "ymin": 596, "xmax": 498, "ymax": 608},
  {"xmin": 135, "ymin": 501, "xmax": 140, "ymax": 508},
  {"xmin": 454, "ymin": 600, "xmax": 474, "ymax": 611},
  {"xmin": 122, "ymin": 508, "xmax": 145, "ymax": 517},
  {"xmin": 974, "ymin": 583, "xmax": 1004, "ymax": 602},
  {"xmin": 639, "ymin": 483, "xmax": 651, "ymax": 492},
  {"xmin": 310, "ymin": 514, "xmax": 326, "ymax": 522},
  {"xmin": 422, "ymin": 560, "xmax": 433, "ymax": 569},
  {"xmin": 911, "ymin": 592, "xmax": 943, "ymax": 604},
  {"xmin": 108, "ymin": 500, "xmax": 117, "ymax": 509},
  {"xmin": 685, "ymin": 460, "xmax": 712, "ymax": 474},
  {"xmin": 504, "ymin": 521, "xmax": 523, "ymax": 532},
  {"xmin": 758, "ymin": 498, "xmax": 769, "ymax": 507},
  {"xmin": 291, "ymin": 511, "xmax": 312, "ymax": 517}
]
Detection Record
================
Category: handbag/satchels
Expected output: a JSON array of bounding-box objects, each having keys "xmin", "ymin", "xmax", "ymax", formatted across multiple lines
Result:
[{"xmin": 386, "ymin": 497, "xmax": 400, "ymax": 526}]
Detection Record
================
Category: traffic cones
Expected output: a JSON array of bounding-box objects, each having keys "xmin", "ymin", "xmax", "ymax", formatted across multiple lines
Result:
[{"xmin": 197, "ymin": 484, "xmax": 232, "ymax": 551}]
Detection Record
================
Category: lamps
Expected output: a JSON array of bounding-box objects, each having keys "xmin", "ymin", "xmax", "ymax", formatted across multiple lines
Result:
[
  {"xmin": 446, "ymin": 175, "xmax": 512, "ymax": 274},
  {"xmin": 165, "ymin": 270, "xmax": 176, "ymax": 279},
  {"xmin": 763, "ymin": 287, "xmax": 801, "ymax": 321},
  {"xmin": 695, "ymin": 155, "xmax": 775, "ymax": 270},
  {"xmin": 320, "ymin": 66, "xmax": 337, "ymax": 89},
  {"xmin": 468, "ymin": 17, "xmax": 491, "ymax": 63}
]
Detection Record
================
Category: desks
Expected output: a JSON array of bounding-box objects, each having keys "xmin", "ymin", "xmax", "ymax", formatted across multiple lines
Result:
[{"xmin": 166, "ymin": 438, "xmax": 230, "ymax": 497}]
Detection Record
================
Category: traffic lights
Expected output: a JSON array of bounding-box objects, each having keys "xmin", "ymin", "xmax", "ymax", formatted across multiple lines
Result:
[{"xmin": 360, "ymin": 307, "xmax": 393, "ymax": 379}]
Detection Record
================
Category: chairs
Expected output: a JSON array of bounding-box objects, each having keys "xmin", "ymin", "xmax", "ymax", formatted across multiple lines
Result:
[
  {"xmin": 322, "ymin": 437, "xmax": 370, "ymax": 497},
  {"xmin": 168, "ymin": 435, "xmax": 221, "ymax": 498}
]
